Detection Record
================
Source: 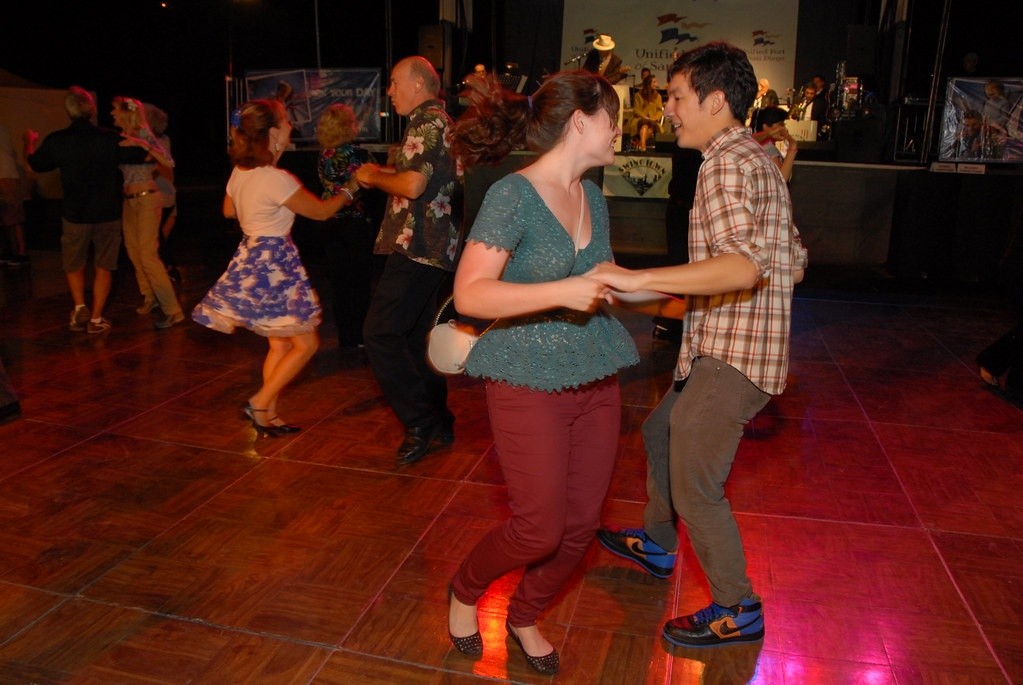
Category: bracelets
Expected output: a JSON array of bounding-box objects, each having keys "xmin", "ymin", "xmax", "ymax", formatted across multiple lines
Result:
[{"xmin": 340, "ymin": 188, "xmax": 353, "ymax": 200}]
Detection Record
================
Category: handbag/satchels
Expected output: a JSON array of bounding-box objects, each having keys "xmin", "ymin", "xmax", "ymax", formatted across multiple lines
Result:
[{"xmin": 428, "ymin": 295, "xmax": 501, "ymax": 374}]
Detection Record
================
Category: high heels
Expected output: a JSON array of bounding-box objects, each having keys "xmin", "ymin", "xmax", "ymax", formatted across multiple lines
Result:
[
  {"xmin": 237, "ymin": 400, "xmax": 286, "ymax": 438},
  {"xmin": 268, "ymin": 416, "xmax": 303, "ymax": 433}
]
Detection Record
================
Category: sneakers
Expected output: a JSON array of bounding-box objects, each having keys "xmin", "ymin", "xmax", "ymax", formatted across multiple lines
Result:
[
  {"xmin": 86, "ymin": 317, "xmax": 112, "ymax": 333},
  {"xmin": 596, "ymin": 526, "xmax": 676, "ymax": 579},
  {"xmin": 69, "ymin": 305, "xmax": 90, "ymax": 332},
  {"xmin": 663, "ymin": 591, "xmax": 765, "ymax": 648}
]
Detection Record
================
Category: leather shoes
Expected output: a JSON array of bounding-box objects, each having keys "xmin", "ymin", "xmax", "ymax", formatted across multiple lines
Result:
[
  {"xmin": 653, "ymin": 320, "xmax": 684, "ymax": 342},
  {"xmin": 436, "ymin": 413, "xmax": 455, "ymax": 447},
  {"xmin": 397, "ymin": 417, "xmax": 444, "ymax": 465}
]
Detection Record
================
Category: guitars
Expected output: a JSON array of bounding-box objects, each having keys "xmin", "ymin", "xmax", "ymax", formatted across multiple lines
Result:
[{"xmin": 604, "ymin": 65, "xmax": 632, "ymax": 79}]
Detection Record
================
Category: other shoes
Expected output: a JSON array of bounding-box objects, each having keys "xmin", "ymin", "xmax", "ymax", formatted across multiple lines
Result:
[
  {"xmin": 0, "ymin": 401, "xmax": 22, "ymax": 424},
  {"xmin": 136, "ymin": 299, "xmax": 160, "ymax": 315},
  {"xmin": 153, "ymin": 311, "xmax": 187, "ymax": 329},
  {"xmin": 974, "ymin": 364, "xmax": 1002, "ymax": 395},
  {"xmin": 506, "ymin": 620, "xmax": 559, "ymax": 675},
  {"xmin": 448, "ymin": 583, "xmax": 484, "ymax": 657}
]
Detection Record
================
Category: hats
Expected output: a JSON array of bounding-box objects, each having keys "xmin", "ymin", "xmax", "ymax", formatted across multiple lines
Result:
[{"xmin": 592, "ymin": 35, "xmax": 616, "ymax": 51}]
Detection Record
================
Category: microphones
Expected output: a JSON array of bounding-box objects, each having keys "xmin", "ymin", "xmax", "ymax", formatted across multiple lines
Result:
[{"xmin": 563, "ymin": 52, "xmax": 589, "ymax": 65}]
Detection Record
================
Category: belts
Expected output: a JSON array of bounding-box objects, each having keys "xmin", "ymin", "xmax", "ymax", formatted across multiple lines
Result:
[{"xmin": 121, "ymin": 188, "xmax": 159, "ymax": 199}]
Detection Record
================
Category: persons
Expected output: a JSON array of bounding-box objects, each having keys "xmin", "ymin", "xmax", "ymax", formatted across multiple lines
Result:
[
  {"xmin": 631, "ymin": 74, "xmax": 662, "ymax": 151},
  {"xmin": 583, "ymin": 36, "xmax": 622, "ymax": 77},
  {"xmin": 317, "ymin": 104, "xmax": 379, "ymax": 366},
  {"xmin": 635, "ymin": 68, "xmax": 659, "ymax": 88},
  {"xmin": 356, "ymin": 56, "xmax": 459, "ymax": 465},
  {"xmin": 954, "ymin": 80, "xmax": 1010, "ymax": 158},
  {"xmin": 797, "ymin": 77, "xmax": 826, "ymax": 121},
  {"xmin": 753, "ymin": 94, "xmax": 772, "ymax": 108},
  {"xmin": 22, "ymin": 85, "xmax": 153, "ymax": 333},
  {"xmin": 652, "ymin": 106, "xmax": 797, "ymax": 339},
  {"xmin": 593, "ymin": 41, "xmax": 809, "ymax": 648},
  {"xmin": 446, "ymin": 70, "xmax": 641, "ymax": 675},
  {"xmin": 193, "ymin": 100, "xmax": 361, "ymax": 439},
  {"xmin": 110, "ymin": 96, "xmax": 186, "ymax": 329}
]
